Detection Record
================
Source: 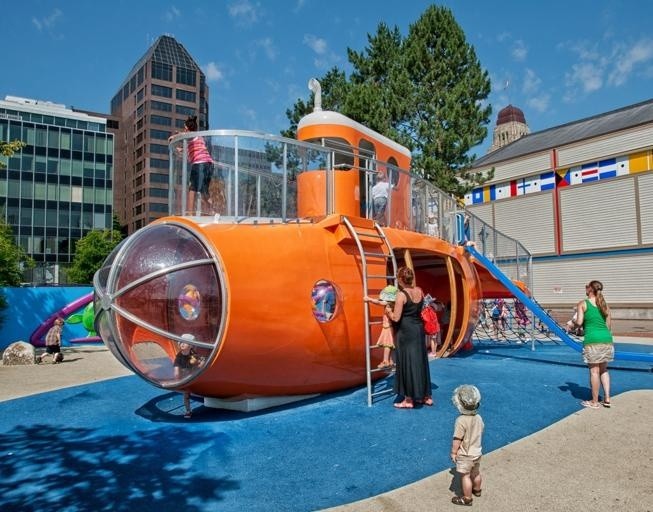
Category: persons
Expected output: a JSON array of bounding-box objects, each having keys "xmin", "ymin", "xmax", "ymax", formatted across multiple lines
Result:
[
  {"xmin": 168, "ymin": 116, "xmax": 214, "ymax": 219},
  {"xmin": 422, "ymin": 211, "xmax": 439, "ymax": 240},
  {"xmin": 449, "ymin": 382, "xmax": 485, "ymax": 506},
  {"xmin": 171, "ymin": 333, "xmax": 205, "ymax": 419},
  {"xmin": 370, "ymin": 171, "xmax": 401, "ymax": 229},
  {"xmin": 461, "ymin": 212, "xmax": 480, "ymax": 254},
  {"xmin": 384, "ymin": 265, "xmax": 434, "ymax": 409},
  {"xmin": 364, "ymin": 285, "xmax": 397, "ymax": 368},
  {"xmin": 476, "ymin": 298, "xmax": 583, "ymax": 345},
  {"xmin": 570, "ymin": 279, "xmax": 615, "ymax": 408},
  {"xmin": 35, "ymin": 317, "xmax": 63, "ymax": 365}
]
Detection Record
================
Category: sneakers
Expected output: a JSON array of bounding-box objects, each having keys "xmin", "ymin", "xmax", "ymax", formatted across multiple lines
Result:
[{"xmin": 183, "ymin": 411, "xmax": 192, "ymax": 418}]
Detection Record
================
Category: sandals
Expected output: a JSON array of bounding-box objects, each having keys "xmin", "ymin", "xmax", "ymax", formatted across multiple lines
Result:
[
  {"xmin": 416, "ymin": 396, "xmax": 433, "ymax": 406},
  {"xmin": 472, "ymin": 488, "xmax": 481, "ymax": 497},
  {"xmin": 377, "ymin": 360, "xmax": 394, "ymax": 368},
  {"xmin": 452, "ymin": 496, "xmax": 473, "ymax": 506},
  {"xmin": 394, "ymin": 399, "xmax": 414, "ymax": 408}
]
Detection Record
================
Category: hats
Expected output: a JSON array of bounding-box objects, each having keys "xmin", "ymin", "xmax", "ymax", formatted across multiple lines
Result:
[
  {"xmin": 451, "ymin": 384, "xmax": 482, "ymax": 416},
  {"xmin": 379, "ymin": 285, "xmax": 400, "ymax": 302},
  {"xmin": 178, "ymin": 334, "xmax": 197, "ymax": 350}
]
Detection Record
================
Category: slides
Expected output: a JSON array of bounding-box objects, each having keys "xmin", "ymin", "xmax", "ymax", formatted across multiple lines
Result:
[{"xmin": 466, "ymin": 245, "xmax": 584, "ymax": 353}]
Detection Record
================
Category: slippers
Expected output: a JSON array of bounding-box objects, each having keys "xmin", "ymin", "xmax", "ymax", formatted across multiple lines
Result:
[
  {"xmin": 601, "ymin": 400, "xmax": 611, "ymax": 407},
  {"xmin": 581, "ymin": 400, "xmax": 600, "ymax": 409}
]
